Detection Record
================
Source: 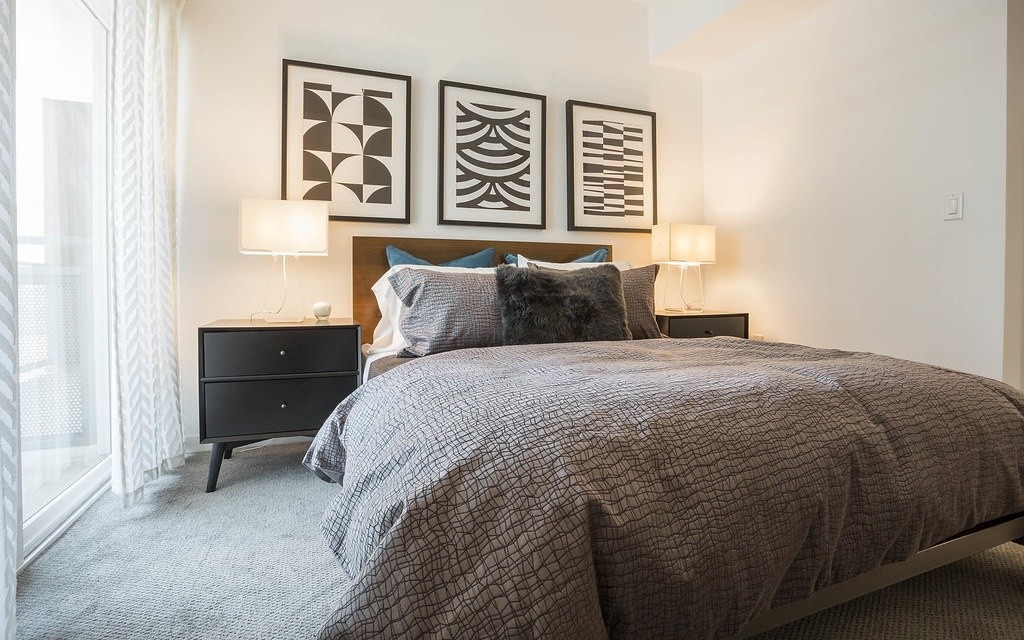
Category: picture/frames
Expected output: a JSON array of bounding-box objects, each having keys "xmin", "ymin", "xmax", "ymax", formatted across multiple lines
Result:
[
  {"xmin": 281, "ymin": 59, "xmax": 411, "ymax": 223},
  {"xmin": 566, "ymin": 99, "xmax": 657, "ymax": 234},
  {"xmin": 437, "ymin": 79, "xmax": 546, "ymax": 229}
]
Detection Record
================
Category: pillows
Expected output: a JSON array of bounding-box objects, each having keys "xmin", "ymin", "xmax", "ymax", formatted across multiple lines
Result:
[
  {"xmin": 503, "ymin": 248, "xmax": 608, "ymax": 263},
  {"xmin": 621, "ymin": 264, "xmax": 668, "ymax": 339},
  {"xmin": 386, "ymin": 245, "xmax": 495, "ymax": 268},
  {"xmin": 517, "ymin": 253, "xmax": 631, "ymax": 271},
  {"xmin": 387, "ymin": 267, "xmax": 502, "ymax": 357},
  {"xmin": 366, "ymin": 264, "xmax": 497, "ymax": 355},
  {"xmin": 493, "ymin": 263, "xmax": 633, "ymax": 345}
]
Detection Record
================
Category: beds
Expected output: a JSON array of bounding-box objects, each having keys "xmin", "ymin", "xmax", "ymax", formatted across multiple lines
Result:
[{"xmin": 301, "ymin": 235, "xmax": 1024, "ymax": 640}]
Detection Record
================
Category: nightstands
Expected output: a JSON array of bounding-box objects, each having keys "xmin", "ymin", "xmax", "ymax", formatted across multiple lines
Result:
[
  {"xmin": 197, "ymin": 318, "xmax": 362, "ymax": 492},
  {"xmin": 654, "ymin": 309, "xmax": 749, "ymax": 339}
]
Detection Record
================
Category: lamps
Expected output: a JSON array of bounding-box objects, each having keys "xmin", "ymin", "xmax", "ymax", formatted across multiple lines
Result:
[
  {"xmin": 237, "ymin": 198, "xmax": 329, "ymax": 320},
  {"xmin": 651, "ymin": 222, "xmax": 716, "ymax": 314}
]
